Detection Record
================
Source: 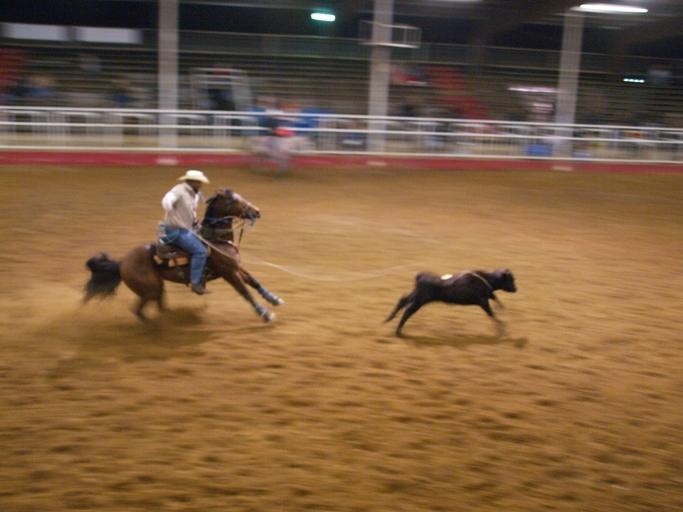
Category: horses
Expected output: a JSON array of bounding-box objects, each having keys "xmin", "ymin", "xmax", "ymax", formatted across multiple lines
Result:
[{"xmin": 82, "ymin": 186, "xmax": 287, "ymax": 324}]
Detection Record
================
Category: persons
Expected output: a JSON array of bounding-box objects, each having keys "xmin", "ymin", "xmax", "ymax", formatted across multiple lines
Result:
[{"xmin": 160, "ymin": 169, "xmax": 209, "ymax": 295}]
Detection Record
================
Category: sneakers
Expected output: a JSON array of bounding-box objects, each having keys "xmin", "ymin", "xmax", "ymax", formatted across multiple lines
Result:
[{"xmin": 191, "ymin": 284, "xmax": 209, "ymax": 294}]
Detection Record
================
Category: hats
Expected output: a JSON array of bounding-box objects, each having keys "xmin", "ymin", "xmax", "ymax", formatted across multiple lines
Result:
[{"xmin": 179, "ymin": 170, "xmax": 210, "ymax": 184}]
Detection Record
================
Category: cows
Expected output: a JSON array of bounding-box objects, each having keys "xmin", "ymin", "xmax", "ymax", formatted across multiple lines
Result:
[{"xmin": 382, "ymin": 269, "xmax": 517, "ymax": 338}]
